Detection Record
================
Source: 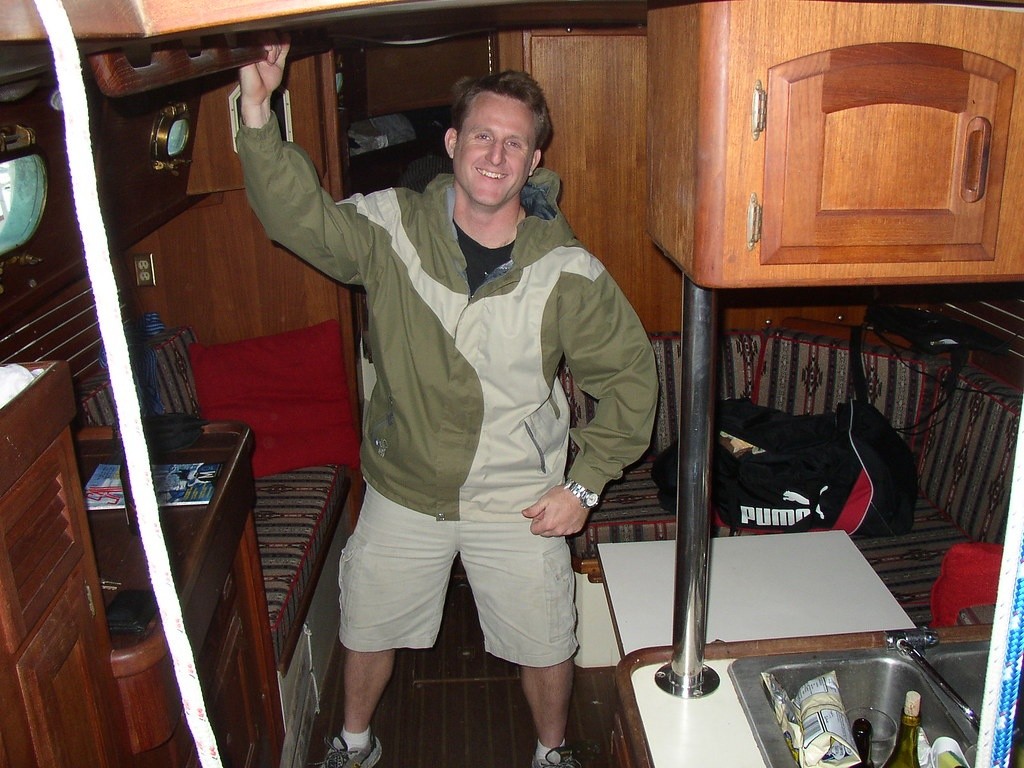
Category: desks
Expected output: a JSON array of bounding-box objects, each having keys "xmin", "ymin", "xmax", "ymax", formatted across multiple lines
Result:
[{"xmin": 594, "ymin": 529, "xmax": 918, "ymax": 660}]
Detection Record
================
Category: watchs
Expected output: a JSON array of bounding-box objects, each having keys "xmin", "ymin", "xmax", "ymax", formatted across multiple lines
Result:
[{"xmin": 564, "ymin": 478, "xmax": 600, "ymax": 508}]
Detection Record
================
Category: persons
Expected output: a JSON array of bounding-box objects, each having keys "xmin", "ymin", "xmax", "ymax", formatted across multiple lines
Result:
[{"xmin": 234, "ymin": 29, "xmax": 660, "ymax": 768}]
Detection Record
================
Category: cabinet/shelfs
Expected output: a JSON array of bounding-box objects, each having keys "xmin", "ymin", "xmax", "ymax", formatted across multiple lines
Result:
[
  {"xmin": 641, "ymin": 0, "xmax": 1024, "ymax": 286},
  {"xmin": 1, "ymin": 359, "xmax": 287, "ymax": 767}
]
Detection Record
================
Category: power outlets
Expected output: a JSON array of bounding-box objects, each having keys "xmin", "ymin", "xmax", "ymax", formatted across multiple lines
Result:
[{"xmin": 133, "ymin": 252, "xmax": 156, "ymax": 286}]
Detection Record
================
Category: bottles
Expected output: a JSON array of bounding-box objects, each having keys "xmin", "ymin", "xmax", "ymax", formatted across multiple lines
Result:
[
  {"xmin": 882, "ymin": 690, "xmax": 921, "ymax": 768},
  {"xmin": 848, "ymin": 718, "xmax": 874, "ymax": 768}
]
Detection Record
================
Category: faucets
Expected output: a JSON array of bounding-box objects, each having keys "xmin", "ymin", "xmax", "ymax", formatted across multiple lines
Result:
[{"xmin": 882, "ymin": 624, "xmax": 982, "ymax": 733}]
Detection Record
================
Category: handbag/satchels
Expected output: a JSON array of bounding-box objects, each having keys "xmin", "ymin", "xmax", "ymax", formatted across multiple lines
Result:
[{"xmin": 652, "ymin": 396, "xmax": 915, "ymax": 538}]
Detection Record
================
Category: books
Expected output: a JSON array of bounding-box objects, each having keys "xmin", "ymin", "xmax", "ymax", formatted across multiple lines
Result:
[{"xmin": 83, "ymin": 464, "xmax": 226, "ymax": 510}]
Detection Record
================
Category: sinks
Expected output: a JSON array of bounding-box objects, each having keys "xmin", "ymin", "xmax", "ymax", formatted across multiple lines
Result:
[
  {"xmin": 725, "ymin": 636, "xmax": 973, "ymax": 768},
  {"xmin": 920, "ymin": 641, "xmax": 990, "ymax": 743}
]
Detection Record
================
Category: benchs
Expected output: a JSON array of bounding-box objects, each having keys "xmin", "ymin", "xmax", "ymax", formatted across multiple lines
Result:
[
  {"xmin": 562, "ymin": 313, "xmax": 1024, "ymax": 669},
  {"xmin": 73, "ymin": 325, "xmax": 354, "ymax": 768}
]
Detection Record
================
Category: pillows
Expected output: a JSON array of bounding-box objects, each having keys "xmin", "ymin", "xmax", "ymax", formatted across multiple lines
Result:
[
  {"xmin": 186, "ymin": 318, "xmax": 360, "ymax": 479},
  {"xmin": 930, "ymin": 540, "xmax": 1005, "ymax": 630}
]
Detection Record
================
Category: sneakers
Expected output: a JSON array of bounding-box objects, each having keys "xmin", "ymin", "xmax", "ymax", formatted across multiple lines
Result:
[
  {"xmin": 531, "ymin": 748, "xmax": 581, "ymax": 768},
  {"xmin": 315, "ymin": 732, "xmax": 382, "ymax": 768}
]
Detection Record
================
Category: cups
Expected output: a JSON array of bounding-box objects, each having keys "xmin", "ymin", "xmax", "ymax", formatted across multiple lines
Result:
[{"xmin": 843, "ymin": 706, "xmax": 898, "ymax": 768}]
